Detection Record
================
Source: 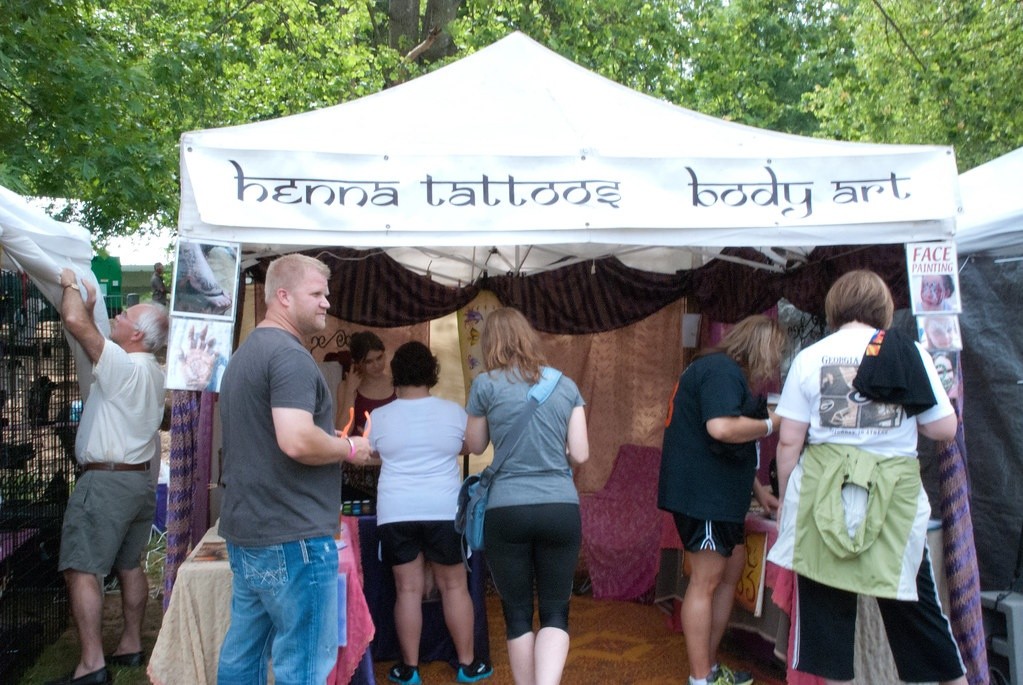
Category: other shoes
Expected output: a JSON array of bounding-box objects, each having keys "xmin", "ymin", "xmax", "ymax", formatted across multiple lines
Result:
[
  {"xmin": 387, "ymin": 662, "xmax": 422, "ymax": 684},
  {"xmin": 44, "ymin": 665, "xmax": 114, "ymax": 685},
  {"xmin": 105, "ymin": 647, "xmax": 146, "ymax": 668},
  {"xmin": 456, "ymin": 657, "xmax": 493, "ymax": 683}
]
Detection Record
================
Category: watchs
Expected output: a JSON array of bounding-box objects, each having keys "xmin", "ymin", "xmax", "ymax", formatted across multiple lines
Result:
[{"xmin": 63, "ymin": 283, "xmax": 81, "ymax": 291}]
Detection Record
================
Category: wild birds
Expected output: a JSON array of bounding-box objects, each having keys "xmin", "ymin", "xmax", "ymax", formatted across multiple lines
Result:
[{"xmin": 44, "ymin": 469, "xmax": 68, "ymax": 503}]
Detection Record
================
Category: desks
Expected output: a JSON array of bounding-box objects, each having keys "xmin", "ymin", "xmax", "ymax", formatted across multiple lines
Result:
[
  {"xmin": 357, "ymin": 515, "xmax": 488, "ymax": 662},
  {"xmin": 146, "ymin": 518, "xmax": 375, "ymax": 685},
  {"xmin": 676, "ymin": 510, "xmax": 951, "ymax": 685}
]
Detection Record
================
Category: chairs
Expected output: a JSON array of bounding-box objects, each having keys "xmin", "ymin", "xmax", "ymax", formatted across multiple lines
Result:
[{"xmin": 577, "ymin": 445, "xmax": 663, "ymax": 604}]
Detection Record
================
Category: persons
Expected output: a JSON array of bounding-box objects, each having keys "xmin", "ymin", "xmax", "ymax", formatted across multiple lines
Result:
[
  {"xmin": 775, "ymin": 269, "xmax": 968, "ymax": 685},
  {"xmin": 916, "ymin": 272, "xmax": 962, "ymax": 399},
  {"xmin": 657, "ymin": 315, "xmax": 791, "ymax": 685},
  {"xmin": 465, "ymin": 306, "xmax": 589, "ymax": 685},
  {"xmin": 176, "ymin": 239, "xmax": 231, "ymax": 388},
  {"xmin": 46, "ymin": 263, "xmax": 169, "ymax": 685},
  {"xmin": 337, "ymin": 330, "xmax": 396, "ymax": 502},
  {"xmin": 365, "ymin": 341, "xmax": 495, "ymax": 685},
  {"xmin": 216, "ymin": 255, "xmax": 371, "ymax": 685}
]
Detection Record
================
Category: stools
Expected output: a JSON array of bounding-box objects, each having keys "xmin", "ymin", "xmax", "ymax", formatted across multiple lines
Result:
[{"xmin": 980, "ymin": 590, "xmax": 1023, "ymax": 685}]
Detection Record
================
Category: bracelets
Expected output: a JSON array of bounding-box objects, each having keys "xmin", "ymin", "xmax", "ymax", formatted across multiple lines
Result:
[
  {"xmin": 764, "ymin": 419, "xmax": 773, "ymax": 438},
  {"xmin": 344, "ymin": 436, "xmax": 356, "ymax": 464}
]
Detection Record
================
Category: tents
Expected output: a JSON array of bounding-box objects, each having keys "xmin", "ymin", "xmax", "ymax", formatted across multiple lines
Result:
[
  {"xmin": 957, "ymin": 146, "xmax": 1023, "ymax": 600},
  {"xmin": 165, "ymin": 31, "xmax": 989, "ymax": 685}
]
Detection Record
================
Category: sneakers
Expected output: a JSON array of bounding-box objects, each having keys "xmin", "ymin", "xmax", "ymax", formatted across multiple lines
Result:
[{"xmin": 688, "ymin": 658, "xmax": 753, "ymax": 685}]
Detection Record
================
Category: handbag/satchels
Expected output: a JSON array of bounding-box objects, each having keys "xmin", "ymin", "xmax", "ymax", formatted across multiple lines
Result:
[{"xmin": 454, "ymin": 471, "xmax": 492, "ymax": 551}]
